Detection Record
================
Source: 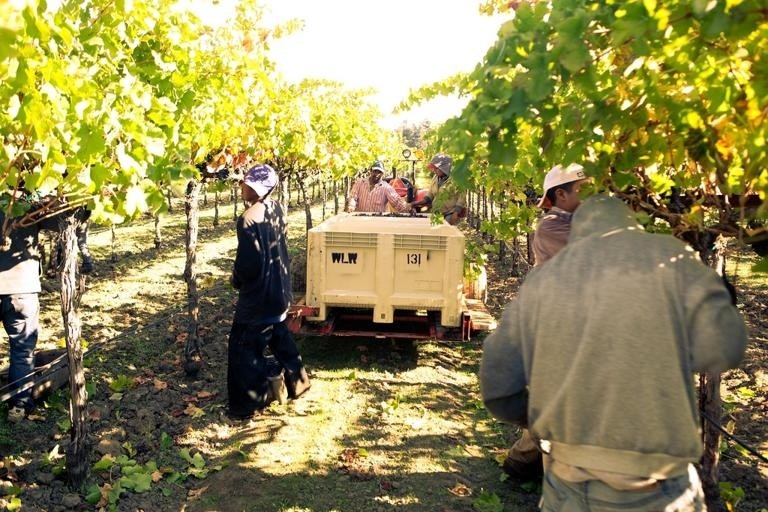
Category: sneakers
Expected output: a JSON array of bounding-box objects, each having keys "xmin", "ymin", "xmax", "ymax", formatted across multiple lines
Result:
[{"xmin": 502, "ymin": 453, "xmax": 546, "ymax": 478}]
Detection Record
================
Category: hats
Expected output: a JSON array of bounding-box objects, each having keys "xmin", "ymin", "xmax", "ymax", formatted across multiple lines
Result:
[
  {"xmin": 240, "ymin": 162, "xmax": 280, "ymax": 199},
  {"xmin": 427, "ymin": 153, "xmax": 454, "ymax": 177},
  {"xmin": 370, "ymin": 161, "xmax": 385, "ymax": 174},
  {"xmin": 535, "ymin": 160, "xmax": 590, "ymax": 211}
]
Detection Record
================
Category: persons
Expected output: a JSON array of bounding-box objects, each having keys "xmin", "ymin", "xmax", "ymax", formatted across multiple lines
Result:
[
  {"xmin": 408, "ymin": 154, "xmax": 469, "ymax": 223},
  {"xmin": 226, "ymin": 161, "xmax": 313, "ymax": 420},
  {"xmin": 343, "ymin": 161, "xmax": 417, "ymax": 213},
  {"xmin": 503, "ymin": 161, "xmax": 596, "ymax": 487},
  {"xmin": 0, "ymin": 161, "xmax": 92, "ymax": 422},
  {"xmin": 472, "ymin": 191, "xmax": 749, "ymax": 511}
]
